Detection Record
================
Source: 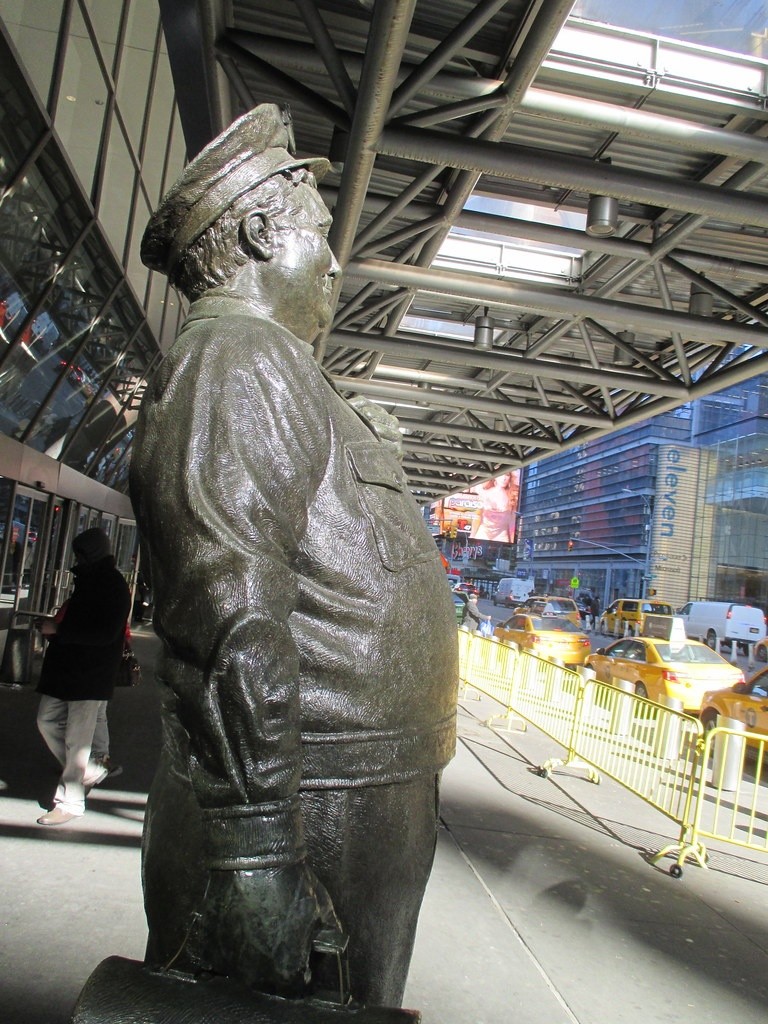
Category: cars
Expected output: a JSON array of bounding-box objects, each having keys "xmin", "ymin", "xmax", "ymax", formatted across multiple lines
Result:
[
  {"xmin": 754, "ymin": 636, "xmax": 768, "ymax": 663},
  {"xmin": 452, "ymin": 583, "xmax": 479, "ymax": 598},
  {"xmin": 583, "ymin": 615, "xmax": 745, "ymax": 718},
  {"xmin": 493, "ymin": 601, "xmax": 592, "ymax": 668},
  {"xmin": 452, "ymin": 591, "xmax": 469, "ymax": 624},
  {"xmin": 698, "ymin": 666, "xmax": 768, "ymax": 766}
]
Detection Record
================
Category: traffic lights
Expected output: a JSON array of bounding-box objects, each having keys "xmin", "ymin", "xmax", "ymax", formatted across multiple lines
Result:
[{"xmin": 568, "ymin": 542, "xmax": 573, "ymax": 551}]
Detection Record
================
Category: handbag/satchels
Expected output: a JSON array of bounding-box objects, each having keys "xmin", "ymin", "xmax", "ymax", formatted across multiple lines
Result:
[{"xmin": 114, "ymin": 640, "xmax": 144, "ymax": 688}]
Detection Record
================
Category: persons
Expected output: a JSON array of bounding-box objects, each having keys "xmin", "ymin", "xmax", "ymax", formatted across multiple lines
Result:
[
  {"xmin": 461, "ymin": 593, "xmax": 494, "ymax": 638},
  {"xmin": 31, "ymin": 526, "xmax": 145, "ymax": 825},
  {"xmin": 68, "ymin": 100, "xmax": 463, "ymax": 1023},
  {"xmin": 528, "ymin": 589, "xmax": 601, "ymax": 630}
]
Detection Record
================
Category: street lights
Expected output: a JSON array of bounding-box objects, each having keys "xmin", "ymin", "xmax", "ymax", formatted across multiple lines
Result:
[
  {"xmin": 623, "ymin": 489, "xmax": 652, "ymax": 599},
  {"xmin": 514, "ymin": 511, "xmax": 535, "ymax": 576}
]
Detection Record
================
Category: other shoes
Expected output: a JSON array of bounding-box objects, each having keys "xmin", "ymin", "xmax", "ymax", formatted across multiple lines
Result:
[
  {"xmin": 84, "ymin": 769, "xmax": 109, "ymax": 796},
  {"xmin": 102, "ymin": 759, "xmax": 123, "ymax": 778},
  {"xmin": 37, "ymin": 807, "xmax": 75, "ymax": 825}
]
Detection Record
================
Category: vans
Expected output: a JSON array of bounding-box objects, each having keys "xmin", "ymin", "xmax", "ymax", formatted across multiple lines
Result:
[
  {"xmin": 674, "ymin": 601, "xmax": 766, "ymax": 656},
  {"xmin": 512, "ymin": 596, "xmax": 582, "ymax": 630},
  {"xmin": 600, "ymin": 598, "xmax": 674, "ymax": 637},
  {"xmin": 493, "ymin": 578, "xmax": 535, "ymax": 608}
]
